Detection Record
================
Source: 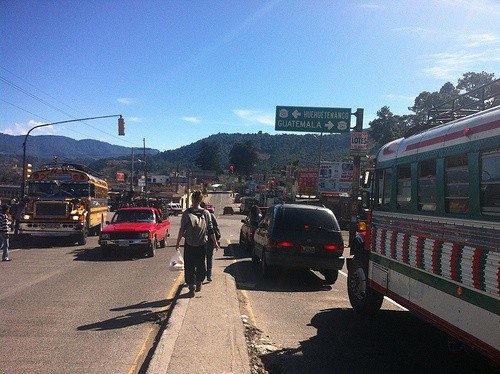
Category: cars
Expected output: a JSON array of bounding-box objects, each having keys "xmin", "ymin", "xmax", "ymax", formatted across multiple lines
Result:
[
  {"xmin": 223, "ymin": 206, "xmax": 234, "ymax": 216},
  {"xmin": 239, "ymin": 205, "xmax": 269, "ymax": 248},
  {"xmin": 206, "ymin": 204, "xmax": 215, "ymax": 214},
  {"xmin": 289, "ymin": 198, "xmax": 326, "ymax": 208}
]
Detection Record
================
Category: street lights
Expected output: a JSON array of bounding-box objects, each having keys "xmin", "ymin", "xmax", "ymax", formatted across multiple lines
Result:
[{"xmin": 20, "ymin": 114, "xmax": 125, "ymax": 196}]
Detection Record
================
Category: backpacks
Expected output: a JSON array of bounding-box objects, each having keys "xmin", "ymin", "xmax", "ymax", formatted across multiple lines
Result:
[{"xmin": 183, "ymin": 208, "xmax": 208, "ymax": 247}]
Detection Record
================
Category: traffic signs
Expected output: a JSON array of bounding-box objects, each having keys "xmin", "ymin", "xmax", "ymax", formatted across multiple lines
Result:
[{"xmin": 275, "ymin": 105, "xmax": 351, "ymax": 133}]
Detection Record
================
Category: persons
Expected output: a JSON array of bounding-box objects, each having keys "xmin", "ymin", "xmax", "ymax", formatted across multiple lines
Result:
[
  {"xmin": 199, "ymin": 202, "xmax": 222, "ymax": 283},
  {"xmin": 1, "ymin": 169, "xmax": 356, "ymax": 263},
  {"xmin": 175, "ymin": 191, "xmax": 220, "ymax": 297}
]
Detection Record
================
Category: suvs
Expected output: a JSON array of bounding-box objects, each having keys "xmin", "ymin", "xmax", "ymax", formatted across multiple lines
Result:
[
  {"xmin": 168, "ymin": 203, "xmax": 183, "ymax": 216},
  {"xmin": 252, "ymin": 201, "xmax": 345, "ymax": 283}
]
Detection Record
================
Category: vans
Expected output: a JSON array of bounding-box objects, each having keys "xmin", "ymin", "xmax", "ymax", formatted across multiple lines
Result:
[{"xmin": 239, "ymin": 198, "xmax": 258, "ymax": 214}]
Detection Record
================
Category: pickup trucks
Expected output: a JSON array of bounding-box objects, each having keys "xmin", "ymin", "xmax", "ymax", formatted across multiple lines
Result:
[{"xmin": 98, "ymin": 207, "xmax": 171, "ymax": 257}]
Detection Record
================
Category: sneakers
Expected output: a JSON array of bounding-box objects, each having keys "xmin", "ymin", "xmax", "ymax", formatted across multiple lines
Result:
[
  {"xmin": 2, "ymin": 257, "xmax": 11, "ymax": 261},
  {"xmin": 188, "ymin": 287, "xmax": 195, "ymax": 297},
  {"xmin": 207, "ymin": 277, "xmax": 212, "ymax": 281},
  {"xmin": 197, "ymin": 282, "xmax": 201, "ymax": 291}
]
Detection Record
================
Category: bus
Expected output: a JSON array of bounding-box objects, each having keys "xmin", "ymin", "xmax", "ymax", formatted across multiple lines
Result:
[
  {"xmin": 18, "ymin": 163, "xmax": 108, "ymax": 245},
  {"xmin": 0, "ymin": 184, "xmax": 29, "ymax": 208},
  {"xmin": 346, "ymin": 77, "xmax": 499, "ymax": 366}
]
